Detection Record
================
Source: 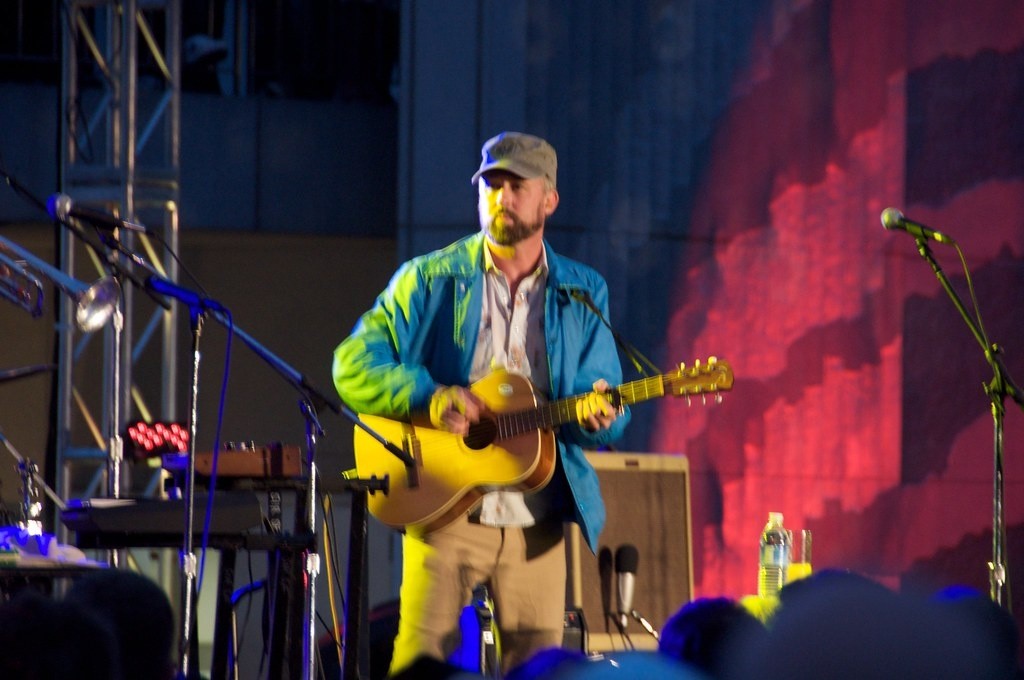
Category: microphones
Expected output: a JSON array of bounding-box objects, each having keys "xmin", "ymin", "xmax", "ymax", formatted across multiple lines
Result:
[
  {"xmin": 881, "ymin": 207, "xmax": 955, "ymax": 245},
  {"xmin": 45, "ymin": 192, "xmax": 156, "ymax": 237},
  {"xmin": 613, "ymin": 542, "xmax": 639, "ymax": 636}
]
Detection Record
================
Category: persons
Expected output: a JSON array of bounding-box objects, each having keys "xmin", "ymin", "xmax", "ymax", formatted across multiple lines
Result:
[
  {"xmin": 514, "ymin": 565, "xmax": 1023, "ymax": 680},
  {"xmin": 333, "ymin": 130, "xmax": 632, "ymax": 680}
]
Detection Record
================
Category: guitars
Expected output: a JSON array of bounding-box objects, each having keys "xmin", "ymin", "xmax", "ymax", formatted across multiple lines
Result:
[{"xmin": 354, "ymin": 355, "xmax": 734, "ymax": 538}]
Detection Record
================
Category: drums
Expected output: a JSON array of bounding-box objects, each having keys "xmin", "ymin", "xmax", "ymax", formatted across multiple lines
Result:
[{"xmin": 0, "ymin": 525, "xmax": 89, "ymax": 560}]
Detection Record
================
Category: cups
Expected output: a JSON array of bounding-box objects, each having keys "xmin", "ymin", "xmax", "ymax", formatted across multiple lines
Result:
[{"xmin": 783, "ymin": 528, "xmax": 812, "ymax": 585}]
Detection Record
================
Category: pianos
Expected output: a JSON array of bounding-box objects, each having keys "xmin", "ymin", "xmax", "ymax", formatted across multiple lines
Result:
[{"xmin": 59, "ymin": 488, "xmax": 307, "ymax": 550}]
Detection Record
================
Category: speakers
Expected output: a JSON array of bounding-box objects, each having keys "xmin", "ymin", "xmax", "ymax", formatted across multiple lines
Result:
[{"xmin": 565, "ymin": 448, "xmax": 692, "ymax": 653}]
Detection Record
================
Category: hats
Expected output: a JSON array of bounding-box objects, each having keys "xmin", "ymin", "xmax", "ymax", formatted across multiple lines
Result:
[{"xmin": 470, "ymin": 132, "xmax": 557, "ymax": 188}]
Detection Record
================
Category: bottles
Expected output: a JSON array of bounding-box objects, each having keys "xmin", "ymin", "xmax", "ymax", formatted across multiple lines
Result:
[{"xmin": 758, "ymin": 511, "xmax": 791, "ymax": 597}]
